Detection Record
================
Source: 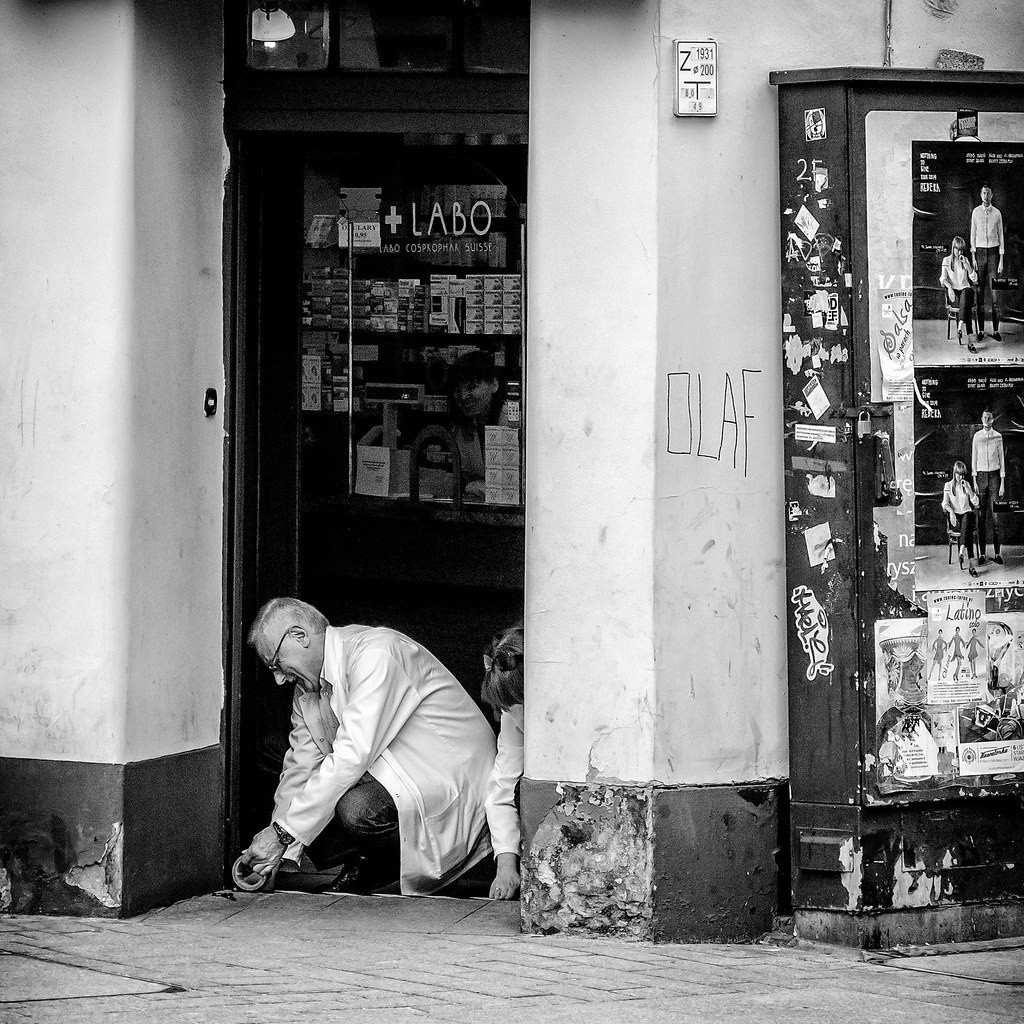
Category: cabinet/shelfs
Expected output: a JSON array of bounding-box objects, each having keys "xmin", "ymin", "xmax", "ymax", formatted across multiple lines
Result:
[
  {"xmin": 299, "ymin": 131, "xmax": 529, "ymax": 736},
  {"xmin": 767, "ymin": 67, "xmax": 1024, "ymax": 952}
]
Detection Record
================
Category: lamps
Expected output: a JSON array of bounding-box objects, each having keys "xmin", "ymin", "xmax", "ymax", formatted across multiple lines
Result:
[{"xmin": 251, "ymin": 7, "xmax": 296, "ymax": 49}]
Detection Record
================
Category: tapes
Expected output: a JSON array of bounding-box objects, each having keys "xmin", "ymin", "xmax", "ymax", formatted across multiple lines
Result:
[{"xmin": 229, "ymin": 855, "xmax": 270, "ymax": 893}]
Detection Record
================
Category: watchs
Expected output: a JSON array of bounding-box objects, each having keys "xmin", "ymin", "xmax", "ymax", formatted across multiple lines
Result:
[{"xmin": 273, "ymin": 821, "xmax": 295, "ymax": 846}]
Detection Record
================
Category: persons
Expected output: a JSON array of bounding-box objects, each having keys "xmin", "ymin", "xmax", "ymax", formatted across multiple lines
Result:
[
  {"xmin": 971, "ymin": 408, "xmax": 1005, "ymax": 566},
  {"xmin": 946, "ymin": 626, "xmax": 968, "ymax": 681},
  {"xmin": 928, "ymin": 628, "xmax": 947, "ymax": 681},
  {"xmin": 938, "ymin": 236, "xmax": 979, "ymax": 354},
  {"xmin": 966, "ymin": 628, "xmax": 985, "ymax": 679},
  {"xmin": 969, "ymin": 185, "xmax": 1005, "ymax": 342},
  {"xmin": 240, "ymin": 597, "xmax": 498, "ymax": 894},
  {"xmin": 447, "ymin": 347, "xmax": 522, "ymax": 498},
  {"xmin": 940, "ymin": 460, "xmax": 979, "ymax": 578},
  {"xmin": 479, "ymin": 620, "xmax": 526, "ymax": 900}
]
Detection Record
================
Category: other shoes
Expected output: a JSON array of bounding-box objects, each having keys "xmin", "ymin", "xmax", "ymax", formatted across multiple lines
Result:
[
  {"xmin": 978, "ymin": 331, "xmax": 984, "ymax": 341},
  {"xmin": 968, "ymin": 344, "xmax": 977, "ymax": 354},
  {"xmin": 957, "ymin": 330, "xmax": 962, "ymax": 338},
  {"xmin": 958, "ymin": 554, "xmax": 964, "ymax": 563},
  {"xmin": 995, "ymin": 555, "xmax": 1003, "ymax": 565},
  {"xmin": 992, "ymin": 331, "xmax": 1002, "ymax": 341},
  {"xmin": 978, "ymin": 555, "xmax": 986, "ymax": 565},
  {"xmin": 969, "ymin": 567, "xmax": 978, "ymax": 578},
  {"xmin": 322, "ymin": 854, "xmax": 379, "ymax": 895}
]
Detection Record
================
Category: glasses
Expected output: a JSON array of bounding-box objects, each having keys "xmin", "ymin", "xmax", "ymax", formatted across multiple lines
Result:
[
  {"xmin": 482, "ymin": 651, "xmax": 524, "ymax": 672},
  {"xmin": 956, "ymin": 247, "xmax": 964, "ymax": 251},
  {"xmin": 957, "ymin": 472, "xmax": 964, "ymax": 476},
  {"xmin": 266, "ymin": 629, "xmax": 289, "ymax": 671}
]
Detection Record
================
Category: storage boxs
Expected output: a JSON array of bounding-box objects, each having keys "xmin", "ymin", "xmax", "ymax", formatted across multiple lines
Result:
[{"xmin": 301, "ymin": 183, "xmax": 525, "ymax": 507}]
